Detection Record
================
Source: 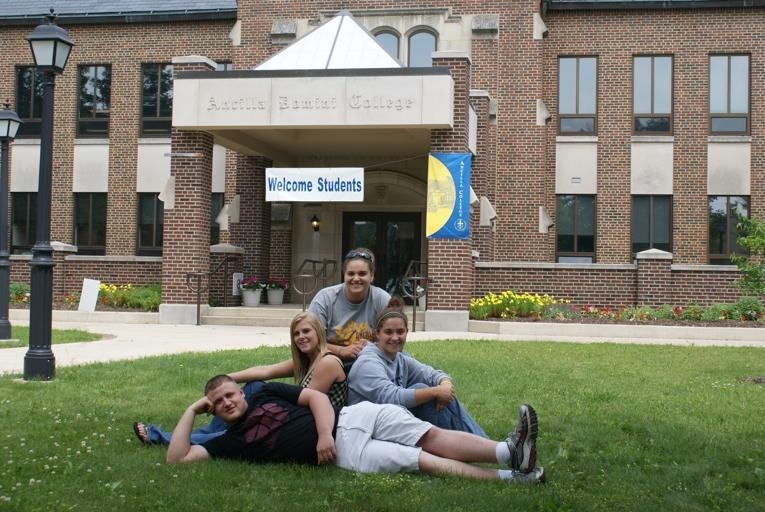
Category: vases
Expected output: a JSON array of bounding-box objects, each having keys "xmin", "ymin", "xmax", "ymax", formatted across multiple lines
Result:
[
  {"xmin": 240, "ymin": 287, "xmax": 262, "ymax": 307},
  {"xmin": 266, "ymin": 288, "xmax": 284, "ymax": 305}
]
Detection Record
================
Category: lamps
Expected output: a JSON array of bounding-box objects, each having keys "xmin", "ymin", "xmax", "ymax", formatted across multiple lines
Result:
[{"xmin": 310, "ymin": 214, "xmax": 321, "ymax": 232}]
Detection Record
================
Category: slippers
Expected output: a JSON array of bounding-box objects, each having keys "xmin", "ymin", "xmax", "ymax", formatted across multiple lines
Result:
[{"xmin": 133, "ymin": 421, "xmax": 148, "ymax": 442}]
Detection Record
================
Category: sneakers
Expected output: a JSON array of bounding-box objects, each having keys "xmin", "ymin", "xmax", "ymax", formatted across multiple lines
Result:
[
  {"xmin": 510, "ymin": 466, "xmax": 547, "ymax": 484},
  {"xmin": 503, "ymin": 399, "xmax": 544, "ymax": 476}
]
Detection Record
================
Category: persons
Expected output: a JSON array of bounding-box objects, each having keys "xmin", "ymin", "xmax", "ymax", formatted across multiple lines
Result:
[
  {"xmin": 308, "ymin": 247, "xmax": 392, "ymax": 374},
  {"xmin": 132, "ymin": 309, "xmax": 349, "ymax": 445},
  {"xmin": 165, "ymin": 373, "xmax": 547, "ymax": 484},
  {"xmin": 347, "ymin": 295, "xmax": 494, "ymax": 439}
]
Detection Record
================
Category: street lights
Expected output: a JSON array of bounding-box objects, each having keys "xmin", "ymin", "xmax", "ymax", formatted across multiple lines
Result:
[
  {"xmin": 21, "ymin": 7, "xmax": 74, "ymax": 381},
  {"xmin": 0, "ymin": 99, "xmax": 21, "ymax": 339}
]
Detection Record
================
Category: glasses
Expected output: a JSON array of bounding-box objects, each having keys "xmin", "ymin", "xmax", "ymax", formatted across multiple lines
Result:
[{"xmin": 343, "ymin": 250, "xmax": 372, "ymax": 261}]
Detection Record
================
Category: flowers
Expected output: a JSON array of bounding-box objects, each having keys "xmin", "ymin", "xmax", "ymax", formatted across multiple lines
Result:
[
  {"xmin": 265, "ymin": 278, "xmax": 287, "ymax": 289},
  {"xmin": 240, "ymin": 275, "xmax": 265, "ymax": 289}
]
